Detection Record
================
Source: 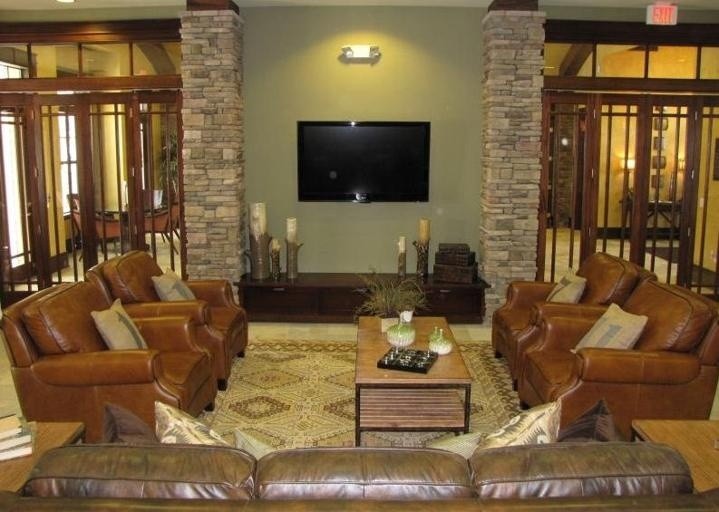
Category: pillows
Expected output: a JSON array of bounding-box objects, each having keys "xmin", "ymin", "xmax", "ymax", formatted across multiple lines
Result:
[
  {"xmin": 547, "ymin": 273, "xmax": 586, "ymax": 303},
  {"xmin": 101, "ymin": 400, "xmax": 280, "ymax": 461},
  {"xmin": 423, "ymin": 397, "xmax": 625, "ymax": 460},
  {"xmin": 570, "ymin": 303, "xmax": 648, "ymax": 355},
  {"xmin": 91, "ymin": 296, "xmax": 147, "ymax": 351},
  {"xmin": 152, "ymin": 266, "xmax": 195, "ymax": 300}
]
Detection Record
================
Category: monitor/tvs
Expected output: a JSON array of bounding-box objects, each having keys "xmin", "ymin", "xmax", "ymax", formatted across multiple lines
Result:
[{"xmin": 296, "ymin": 120, "xmax": 430, "ymax": 202}]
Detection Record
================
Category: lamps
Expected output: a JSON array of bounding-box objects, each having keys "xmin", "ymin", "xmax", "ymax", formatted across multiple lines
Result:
[{"xmin": 340, "ymin": 46, "xmax": 379, "ymax": 61}]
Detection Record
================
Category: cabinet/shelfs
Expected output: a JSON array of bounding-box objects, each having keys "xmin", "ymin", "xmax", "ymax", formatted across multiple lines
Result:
[{"xmin": 233, "ymin": 272, "xmax": 491, "ymax": 325}]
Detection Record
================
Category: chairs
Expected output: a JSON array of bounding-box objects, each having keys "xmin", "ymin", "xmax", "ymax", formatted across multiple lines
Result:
[
  {"xmin": 85, "ymin": 249, "xmax": 249, "ymax": 390},
  {"xmin": 0, "ymin": 281, "xmax": 217, "ymax": 444},
  {"xmin": 491, "ymin": 253, "xmax": 656, "ymax": 392},
  {"xmin": 519, "ymin": 278, "xmax": 719, "ymax": 441},
  {"xmin": 66, "ymin": 194, "xmax": 181, "ymax": 261}
]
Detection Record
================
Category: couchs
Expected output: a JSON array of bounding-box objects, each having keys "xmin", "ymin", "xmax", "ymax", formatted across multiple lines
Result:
[{"xmin": 1, "ymin": 434, "xmax": 719, "ymax": 512}]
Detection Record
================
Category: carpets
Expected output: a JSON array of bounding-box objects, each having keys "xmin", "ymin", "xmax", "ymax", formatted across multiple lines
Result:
[{"xmin": 195, "ymin": 338, "xmax": 520, "ymax": 449}]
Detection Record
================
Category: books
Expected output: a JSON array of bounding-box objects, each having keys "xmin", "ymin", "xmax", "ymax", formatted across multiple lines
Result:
[{"xmin": 0, "ymin": 412, "xmax": 33, "ymax": 463}]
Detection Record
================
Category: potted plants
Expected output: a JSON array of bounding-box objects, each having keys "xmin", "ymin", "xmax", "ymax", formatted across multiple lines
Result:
[{"xmin": 354, "ymin": 267, "xmax": 431, "ymax": 333}]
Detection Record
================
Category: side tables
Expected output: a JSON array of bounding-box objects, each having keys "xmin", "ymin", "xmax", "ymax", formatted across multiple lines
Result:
[
  {"xmin": 631, "ymin": 417, "xmax": 719, "ymax": 491},
  {"xmin": 1, "ymin": 420, "xmax": 88, "ymax": 491}
]
